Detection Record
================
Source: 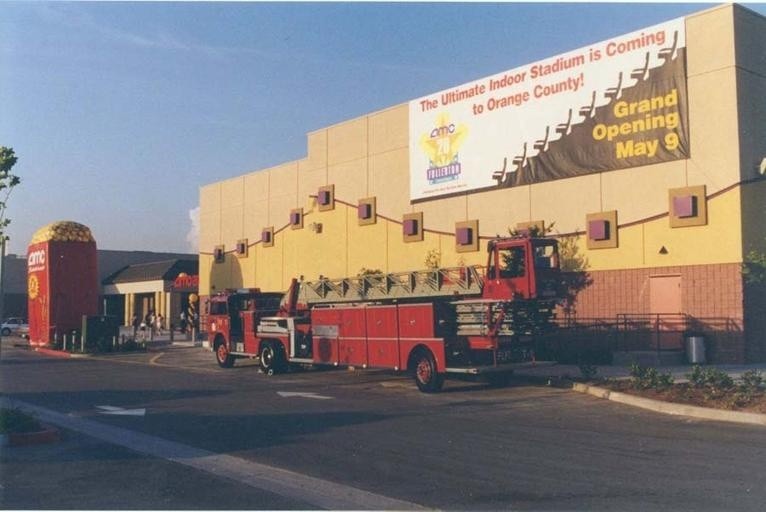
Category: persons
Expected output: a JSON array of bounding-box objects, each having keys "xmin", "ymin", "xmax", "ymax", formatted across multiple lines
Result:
[{"xmin": 131, "ymin": 311, "xmax": 199, "ymax": 340}]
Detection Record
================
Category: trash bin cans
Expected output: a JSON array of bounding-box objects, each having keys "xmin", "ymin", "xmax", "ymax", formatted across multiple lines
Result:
[
  {"xmin": 81, "ymin": 314, "xmax": 120, "ymax": 354},
  {"xmin": 684, "ymin": 331, "xmax": 707, "ymax": 365}
]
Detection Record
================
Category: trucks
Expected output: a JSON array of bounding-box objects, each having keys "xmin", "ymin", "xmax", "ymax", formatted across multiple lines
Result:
[{"xmin": 205, "ymin": 237, "xmax": 567, "ymax": 392}]
